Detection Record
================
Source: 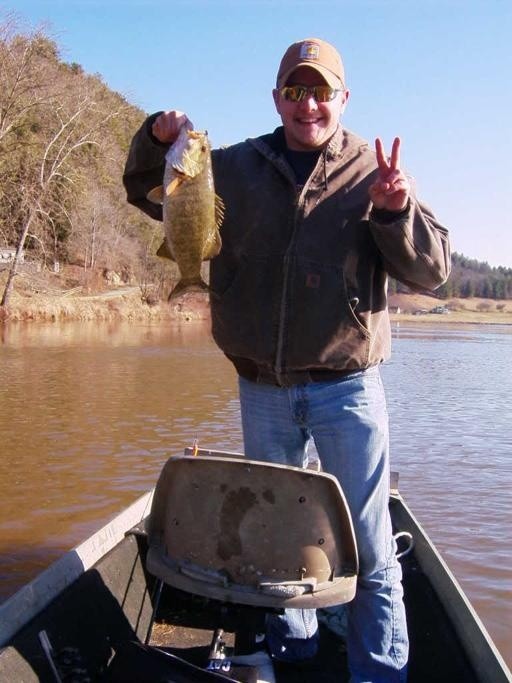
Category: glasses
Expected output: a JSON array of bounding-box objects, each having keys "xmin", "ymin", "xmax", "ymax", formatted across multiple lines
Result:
[{"xmin": 279, "ymin": 84, "xmax": 338, "ymax": 103}]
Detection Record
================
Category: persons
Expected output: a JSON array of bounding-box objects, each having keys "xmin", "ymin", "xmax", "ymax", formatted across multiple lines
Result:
[{"xmin": 120, "ymin": 34, "xmax": 452, "ymax": 681}]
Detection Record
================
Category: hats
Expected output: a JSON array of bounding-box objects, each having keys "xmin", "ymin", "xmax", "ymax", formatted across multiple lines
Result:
[{"xmin": 275, "ymin": 36, "xmax": 347, "ymax": 90}]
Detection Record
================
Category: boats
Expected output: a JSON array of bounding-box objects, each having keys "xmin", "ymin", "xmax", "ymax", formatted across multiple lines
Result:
[{"xmin": 2, "ymin": 446, "xmax": 511, "ymax": 682}]
